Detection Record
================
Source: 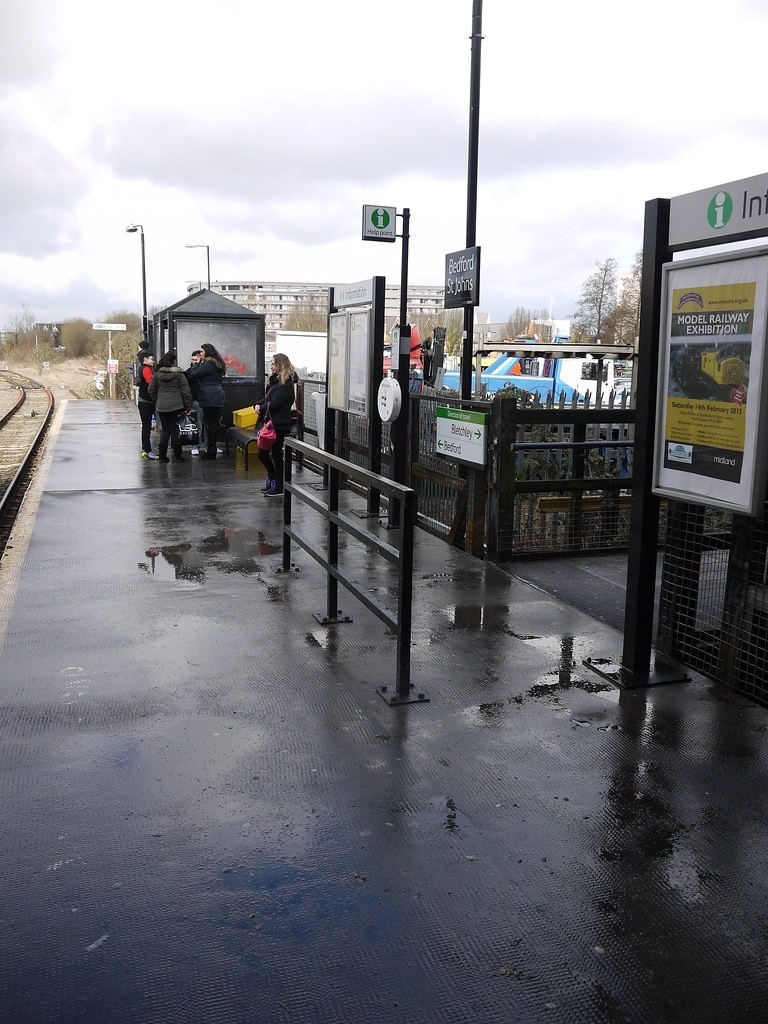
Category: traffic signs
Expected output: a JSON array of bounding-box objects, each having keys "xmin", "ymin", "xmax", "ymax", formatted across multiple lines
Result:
[{"xmin": 436, "ymin": 405, "xmax": 489, "ymax": 471}]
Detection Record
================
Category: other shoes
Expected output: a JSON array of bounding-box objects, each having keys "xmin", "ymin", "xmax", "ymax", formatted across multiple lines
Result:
[
  {"xmin": 159, "ymin": 457, "xmax": 169, "ymax": 463},
  {"xmin": 176, "ymin": 457, "xmax": 185, "ymax": 463},
  {"xmin": 141, "ymin": 452, "xmax": 155, "ymax": 457},
  {"xmin": 191, "ymin": 449, "xmax": 199, "ymax": 454},
  {"xmin": 144, "ymin": 453, "xmax": 159, "ymax": 460},
  {"xmin": 264, "ymin": 488, "xmax": 284, "ymax": 496},
  {"xmin": 260, "ymin": 486, "xmax": 270, "ymax": 493},
  {"xmin": 206, "ymin": 448, "xmax": 224, "ymax": 454}
]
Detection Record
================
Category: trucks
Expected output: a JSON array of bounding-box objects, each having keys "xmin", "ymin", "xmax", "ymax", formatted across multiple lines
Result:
[{"xmin": 411, "ymin": 334, "xmax": 616, "ymax": 406}]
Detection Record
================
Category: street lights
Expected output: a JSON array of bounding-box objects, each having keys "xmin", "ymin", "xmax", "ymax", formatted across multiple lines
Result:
[
  {"xmin": 125, "ymin": 222, "xmax": 149, "ymax": 343},
  {"xmin": 185, "ymin": 244, "xmax": 211, "ymax": 291}
]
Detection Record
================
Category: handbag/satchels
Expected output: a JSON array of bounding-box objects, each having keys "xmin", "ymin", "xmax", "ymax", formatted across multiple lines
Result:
[
  {"xmin": 176, "ymin": 410, "xmax": 200, "ymax": 445},
  {"xmin": 257, "ymin": 402, "xmax": 276, "ymax": 451}
]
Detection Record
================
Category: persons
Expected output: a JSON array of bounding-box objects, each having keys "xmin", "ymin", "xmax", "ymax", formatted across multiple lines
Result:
[
  {"xmin": 186, "ymin": 349, "xmax": 224, "ymax": 455},
  {"xmin": 147, "ymin": 352, "xmax": 193, "ymax": 463},
  {"xmin": 189, "ymin": 343, "xmax": 226, "ymax": 461},
  {"xmin": 253, "ymin": 353, "xmax": 300, "ymax": 497},
  {"xmin": 137, "ymin": 352, "xmax": 160, "ymax": 460},
  {"xmin": 134, "ymin": 341, "xmax": 157, "ymax": 430}
]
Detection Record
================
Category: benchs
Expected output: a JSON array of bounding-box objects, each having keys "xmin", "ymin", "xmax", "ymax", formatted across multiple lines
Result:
[{"xmin": 224, "ymin": 425, "xmax": 265, "ymax": 470}]
